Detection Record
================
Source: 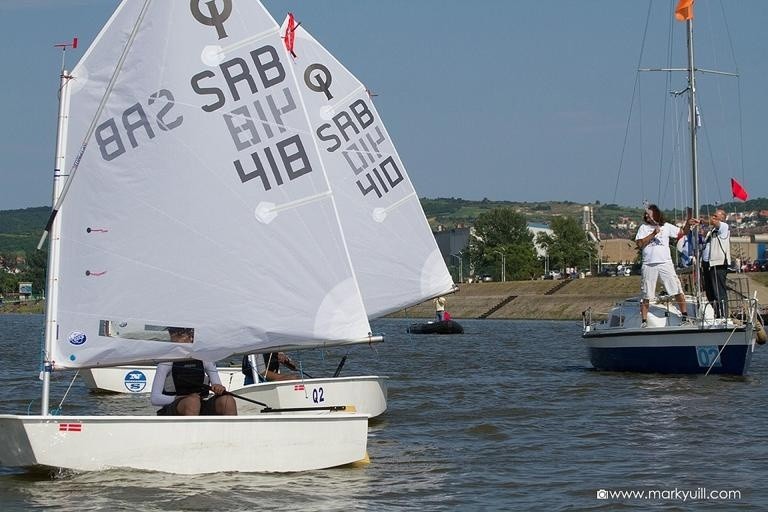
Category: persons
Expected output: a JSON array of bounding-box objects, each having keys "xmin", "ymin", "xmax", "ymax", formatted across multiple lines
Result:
[
  {"xmin": 150, "ymin": 326, "xmax": 237, "ymax": 416},
  {"xmin": 432, "ymin": 296, "xmax": 451, "ymax": 322},
  {"xmin": 242, "ymin": 351, "xmax": 302, "ymax": 385},
  {"xmin": 675, "ymin": 209, "xmax": 731, "ymax": 328},
  {"xmin": 633, "ymin": 204, "xmax": 696, "ymax": 328}
]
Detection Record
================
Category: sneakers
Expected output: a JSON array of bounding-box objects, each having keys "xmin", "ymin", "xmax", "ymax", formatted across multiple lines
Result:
[{"xmin": 642, "ymin": 322, "xmax": 647, "ymax": 327}]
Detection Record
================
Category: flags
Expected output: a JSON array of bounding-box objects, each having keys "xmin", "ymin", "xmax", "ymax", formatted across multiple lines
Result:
[{"xmin": 731, "ymin": 178, "xmax": 747, "ymax": 202}]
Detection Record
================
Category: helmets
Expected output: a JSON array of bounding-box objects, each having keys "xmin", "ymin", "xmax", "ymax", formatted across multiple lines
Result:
[{"xmin": 164, "ymin": 328, "xmax": 195, "ymax": 336}]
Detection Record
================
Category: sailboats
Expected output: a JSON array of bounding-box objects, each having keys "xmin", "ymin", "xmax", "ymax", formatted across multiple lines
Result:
[{"xmin": 584, "ymin": 0, "xmax": 768, "ymax": 377}]
